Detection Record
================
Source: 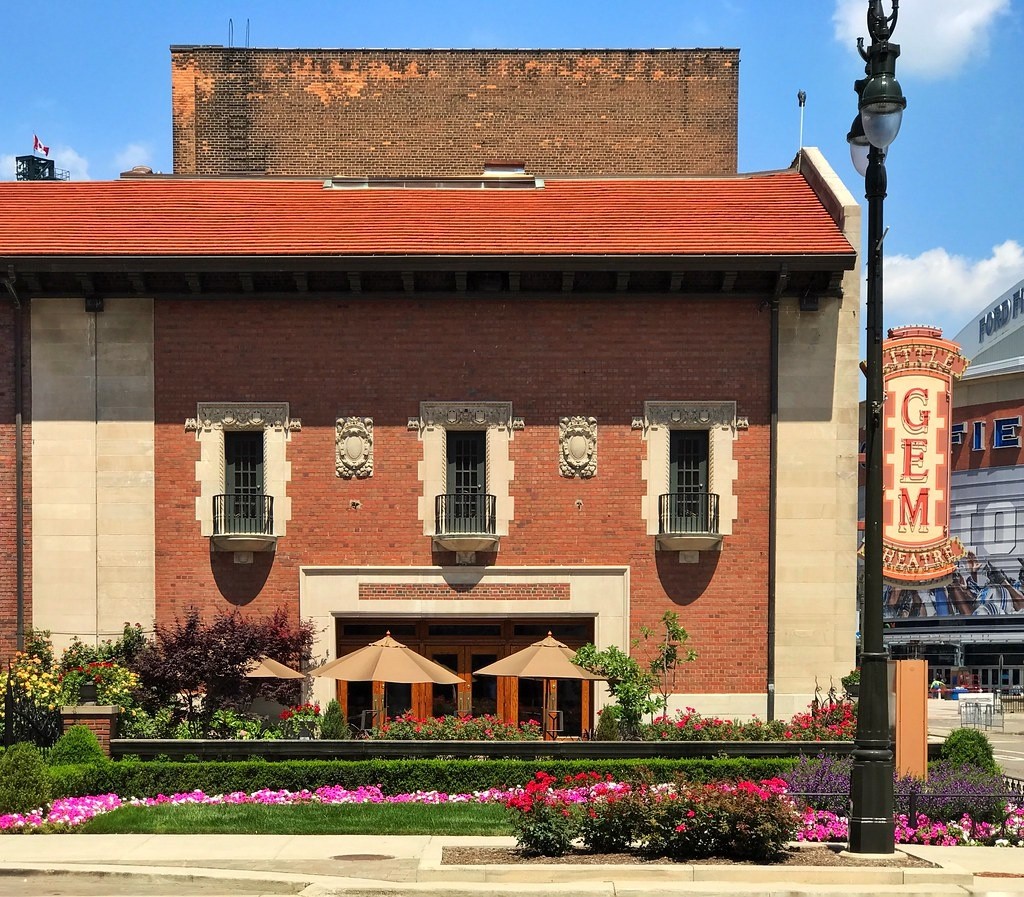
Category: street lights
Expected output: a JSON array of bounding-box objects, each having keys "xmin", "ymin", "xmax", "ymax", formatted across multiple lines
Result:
[{"xmin": 851, "ymin": 0, "xmax": 898, "ymax": 850}]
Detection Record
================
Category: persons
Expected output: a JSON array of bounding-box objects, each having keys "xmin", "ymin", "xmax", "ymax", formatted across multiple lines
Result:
[{"xmin": 930, "ymin": 676, "xmax": 947, "ymax": 698}]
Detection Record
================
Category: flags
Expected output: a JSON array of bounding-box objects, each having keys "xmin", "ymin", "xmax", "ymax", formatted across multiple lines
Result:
[{"xmin": 32, "ymin": 133, "xmax": 51, "ymax": 156}]
